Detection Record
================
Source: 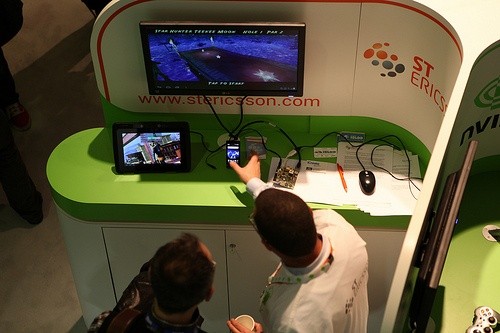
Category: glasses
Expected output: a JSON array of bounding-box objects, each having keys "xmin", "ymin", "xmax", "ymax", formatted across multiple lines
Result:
[{"xmin": 248, "ymin": 212, "xmax": 263, "ymax": 240}]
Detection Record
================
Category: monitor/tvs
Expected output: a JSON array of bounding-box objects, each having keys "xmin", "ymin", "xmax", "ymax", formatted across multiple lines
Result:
[{"xmin": 138, "ymin": 21, "xmax": 306, "ymax": 97}]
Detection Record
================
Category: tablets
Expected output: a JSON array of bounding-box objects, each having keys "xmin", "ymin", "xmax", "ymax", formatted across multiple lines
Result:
[{"xmin": 112, "ymin": 122, "xmax": 192, "ymax": 173}]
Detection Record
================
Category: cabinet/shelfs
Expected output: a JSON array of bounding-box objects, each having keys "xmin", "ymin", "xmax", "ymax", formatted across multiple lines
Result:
[{"xmin": 45, "ymin": 128, "xmax": 423, "ymax": 333}]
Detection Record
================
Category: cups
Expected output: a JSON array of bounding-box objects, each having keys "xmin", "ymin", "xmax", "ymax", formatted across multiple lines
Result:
[{"xmin": 234, "ymin": 315, "xmax": 256, "ymax": 333}]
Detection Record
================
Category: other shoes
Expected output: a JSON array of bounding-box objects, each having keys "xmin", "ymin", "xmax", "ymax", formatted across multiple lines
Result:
[
  {"xmin": 9, "ymin": 202, "xmax": 44, "ymax": 225},
  {"xmin": 7, "ymin": 102, "xmax": 32, "ymax": 131}
]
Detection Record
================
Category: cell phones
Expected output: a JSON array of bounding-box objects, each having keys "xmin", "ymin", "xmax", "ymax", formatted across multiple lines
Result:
[{"xmin": 226, "ymin": 140, "xmax": 241, "ymax": 168}]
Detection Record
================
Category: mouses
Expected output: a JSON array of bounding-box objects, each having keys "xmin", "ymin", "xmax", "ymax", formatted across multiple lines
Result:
[{"xmin": 359, "ymin": 170, "xmax": 376, "ymax": 194}]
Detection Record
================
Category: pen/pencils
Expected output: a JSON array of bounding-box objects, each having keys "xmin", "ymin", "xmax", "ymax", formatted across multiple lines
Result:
[{"xmin": 337, "ymin": 163, "xmax": 348, "ymax": 193}]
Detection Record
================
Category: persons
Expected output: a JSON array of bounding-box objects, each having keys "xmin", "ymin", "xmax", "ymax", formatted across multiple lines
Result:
[
  {"xmin": 0, "ymin": 0, "xmax": 46, "ymax": 225},
  {"xmin": 85, "ymin": 230, "xmax": 218, "ymax": 333},
  {"xmin": 226, "ymin": 152, "xmax": 369, "ymax": 333}
]
